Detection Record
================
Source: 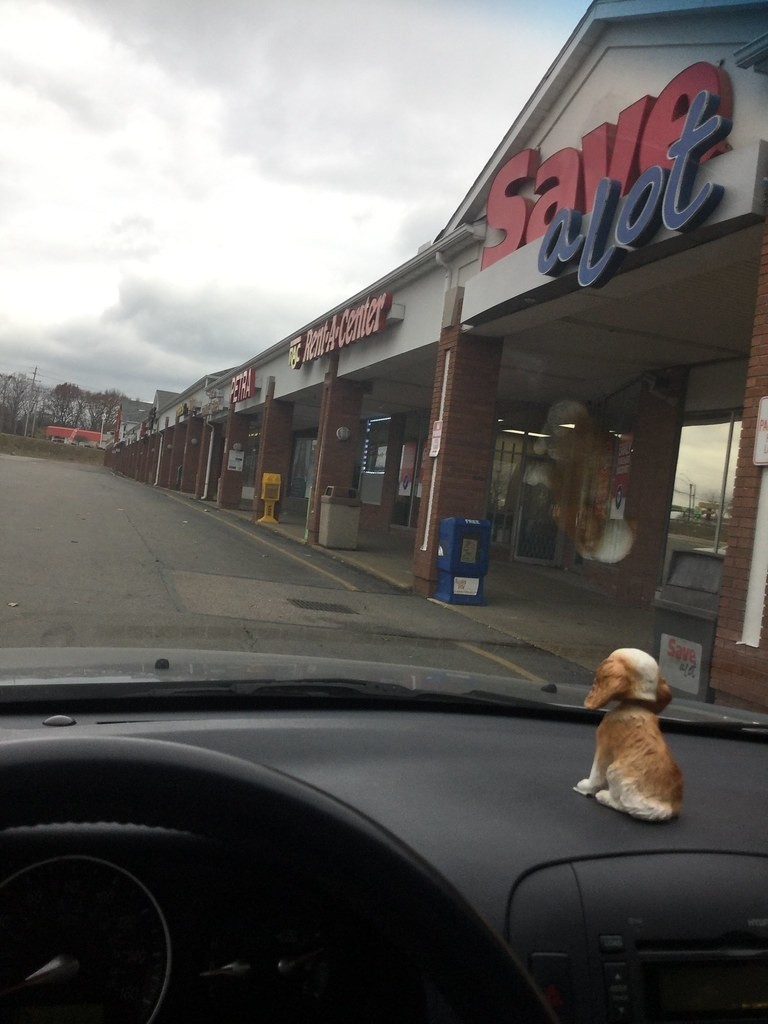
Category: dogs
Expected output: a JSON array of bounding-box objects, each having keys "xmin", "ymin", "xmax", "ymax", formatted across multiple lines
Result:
[{"xmin": 572, "ymin": 647, "xmax": 684, "ymax": 822}]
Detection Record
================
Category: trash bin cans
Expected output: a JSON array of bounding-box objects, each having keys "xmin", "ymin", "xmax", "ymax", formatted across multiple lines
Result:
[
  {"xmin": 651, "ymin": 545, "xmax": 727, "ymax": 705},
  {"xmin": 318, "ymin": 486, "xmax": 362, "ymax": 550}
]
